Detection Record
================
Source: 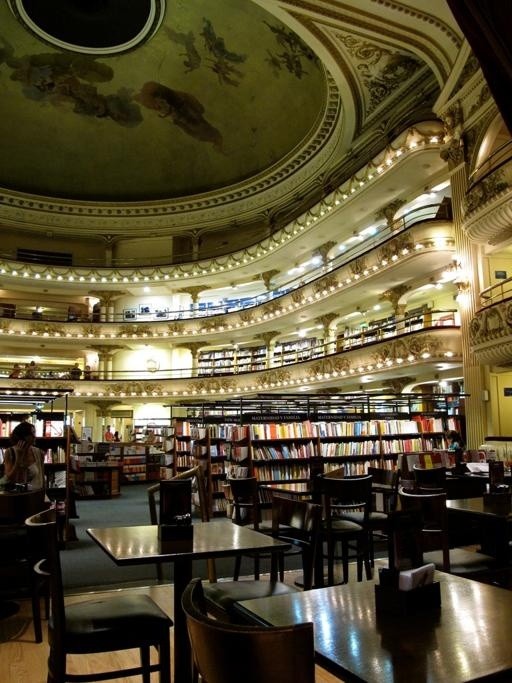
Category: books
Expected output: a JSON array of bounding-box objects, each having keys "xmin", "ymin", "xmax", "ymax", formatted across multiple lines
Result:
[
  {"xmin": 0, "ymin": 418, "xmax": 68, "ymax": 463},
  {"xmin": 43, "ymin": 463, "xmax": 67, "ymax": 513},
  {"xmin": 70, "ymin": 425, "xmax": 159, "ymax": 497}
]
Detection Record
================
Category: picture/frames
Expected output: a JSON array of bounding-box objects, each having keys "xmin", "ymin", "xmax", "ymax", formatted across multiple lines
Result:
[{"xmin": 122, "ymin": 302, "xmax": 169, "ymax": 320}]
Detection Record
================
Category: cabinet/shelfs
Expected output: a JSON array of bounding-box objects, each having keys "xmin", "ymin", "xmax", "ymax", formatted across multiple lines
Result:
[
  {"xmin": 170, "ymin": 400, "xmax": 463, "ymax": 513},
  {"xmin": 196, "ymin": 335, "xmax": 324, "ymax": 376},
  {"xmin": 70, "ymin": 416, "xmax": 170, "ymax": 500},
  {"xmin": 335, "ymin": 302, "xmax": 432, "ymax": 352},
  {"xmin": 1, "ymin": 412, "xmax": 76, "ymax": 513}
]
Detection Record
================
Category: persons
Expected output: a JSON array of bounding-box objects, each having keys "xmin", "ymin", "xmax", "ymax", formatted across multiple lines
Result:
[
  {"xmin": 9, "ymin": 363, "xmax": 22, "ymax": 379},
  {"xmin": 25, "ymin": 360, "xmax": 36, "ymax": 378},
  {"xmin": 4, "ymin": 421, "xmax": 46, "ymax": 502},
  {"xmin": 84, "ymin": 364, "xmax": 91, "ymax": 379},
  {"xmin": 46, "ymin": 370, "xmax": 56, "ymax": 378},
  {"xmin": 69, "ymin": 362, "xmax": 82, "ymax": 379}
]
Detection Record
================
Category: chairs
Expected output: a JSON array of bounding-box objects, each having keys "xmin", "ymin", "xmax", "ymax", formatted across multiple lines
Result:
[{"xmin": 0, "ymin": 469, "xmax": 511, "ymax": 683}]
